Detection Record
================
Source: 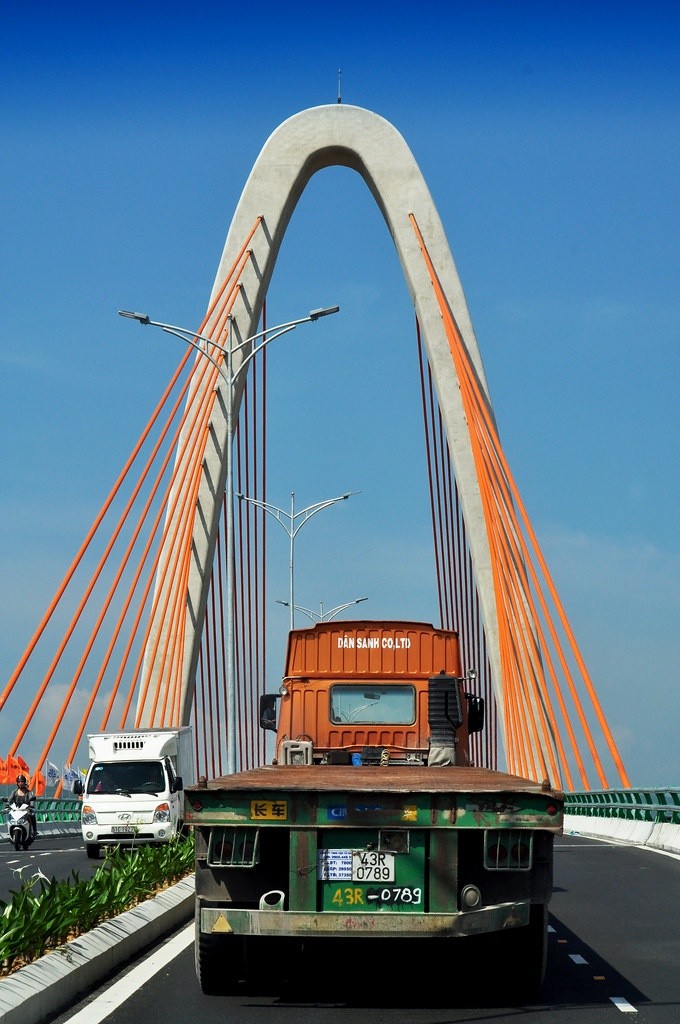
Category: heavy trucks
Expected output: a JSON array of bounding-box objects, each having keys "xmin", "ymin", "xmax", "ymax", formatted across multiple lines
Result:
[{"xmin": 178, "ymin": 618, "xmax": 568, "ymax": 999}]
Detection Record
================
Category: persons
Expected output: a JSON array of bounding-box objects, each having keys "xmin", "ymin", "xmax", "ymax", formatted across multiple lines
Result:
[
  {"xmin": 142, "ymin": 767, "xmax": 163, "ymax": 787},
  {"xmin": 5, "ymin": 774, "xmax": 39, "ymax": 837}
]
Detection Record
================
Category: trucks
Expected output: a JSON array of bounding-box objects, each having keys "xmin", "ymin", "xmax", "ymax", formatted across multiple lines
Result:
[{"xmin": 78, "ymin": 726, "xmax": 196, "ymax": 859}]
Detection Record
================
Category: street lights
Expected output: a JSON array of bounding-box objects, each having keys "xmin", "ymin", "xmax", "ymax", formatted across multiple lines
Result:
[
  {"xmin": 232, "ymin": 489, "xmax": 362, "ymax": 630},
  {"xmin": 119, "ymin": 303, "xmax": 341, "ymax": 774}
]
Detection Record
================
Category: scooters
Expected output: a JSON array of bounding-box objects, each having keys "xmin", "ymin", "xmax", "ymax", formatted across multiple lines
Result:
[{"xmin": 0, "ymin": 796, "xmax": 38, "ymax": 851}]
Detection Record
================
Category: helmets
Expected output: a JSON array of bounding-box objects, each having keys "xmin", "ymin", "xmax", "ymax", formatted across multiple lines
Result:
[{"xmin": 17, "ymin": 775, "xmax": 26, "ymax": 786}]
